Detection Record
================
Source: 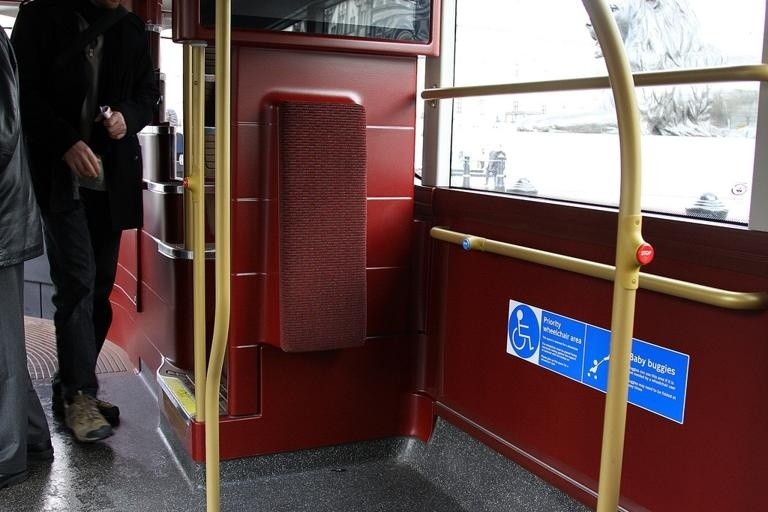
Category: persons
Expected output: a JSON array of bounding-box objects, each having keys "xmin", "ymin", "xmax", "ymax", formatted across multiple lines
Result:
[
  {"xmin": 14, "ymin": 2, "xmax": 166, "ymax": 444},
  {"xmin": 0, "ymin": 25, "xmax": 56, "ymax": 488}
]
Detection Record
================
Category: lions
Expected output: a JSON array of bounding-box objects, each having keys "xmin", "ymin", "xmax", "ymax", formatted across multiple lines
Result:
[{"xmin": 583, "ymin": 0, "xmax": 734, "ymax": 137}]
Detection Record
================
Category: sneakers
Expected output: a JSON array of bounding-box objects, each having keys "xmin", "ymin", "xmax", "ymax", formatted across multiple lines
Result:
[
  {"xmin": 52, "ymin": 384, "xmax": 120, "ymax": 421},
  {"xmin": 64, "ymin": 392, "xmax": 115, "ymax": 445},
  {"xmin": 0, "ymin": 464, "xmax": 32, "ymax": 489},
  {"xmin": 26, "ymin": 436, "xmax": 54, "ymax": 461}
]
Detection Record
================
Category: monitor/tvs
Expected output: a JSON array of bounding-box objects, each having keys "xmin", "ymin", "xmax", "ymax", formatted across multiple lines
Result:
[{"xmin": 172, "ymin": 0, "xmax": 441, "ymax": 56}]
